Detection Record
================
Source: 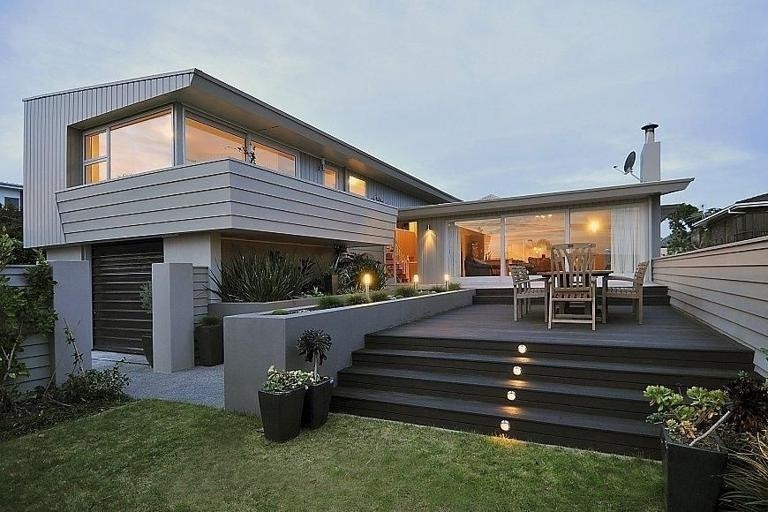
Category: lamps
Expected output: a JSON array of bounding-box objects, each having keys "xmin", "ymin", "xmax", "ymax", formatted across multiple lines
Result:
[{"xmin": 425, "ymin": 224, "xmax": 433, "ymax": 232}]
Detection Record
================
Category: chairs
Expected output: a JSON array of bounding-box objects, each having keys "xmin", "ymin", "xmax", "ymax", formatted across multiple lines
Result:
[{"xmin": 507, "ymin": 243, "xmax": 651, "ymax": 330}]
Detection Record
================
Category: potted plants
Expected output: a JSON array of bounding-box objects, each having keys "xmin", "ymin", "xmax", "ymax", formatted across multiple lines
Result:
[
  {"xmin": 193, "ymin": 313, "xmax": 223, "ymax": 366},
  {"xmin": 137, "ymin": 281, "xmax": 153, "ymax": 369},
  {"xmin": 257, "ymin": 328, "xmax": 335, "ymax": 443},
  {"xmin": 644, "ymin": 382, "xmax": 732, "ymax": 512}
]
